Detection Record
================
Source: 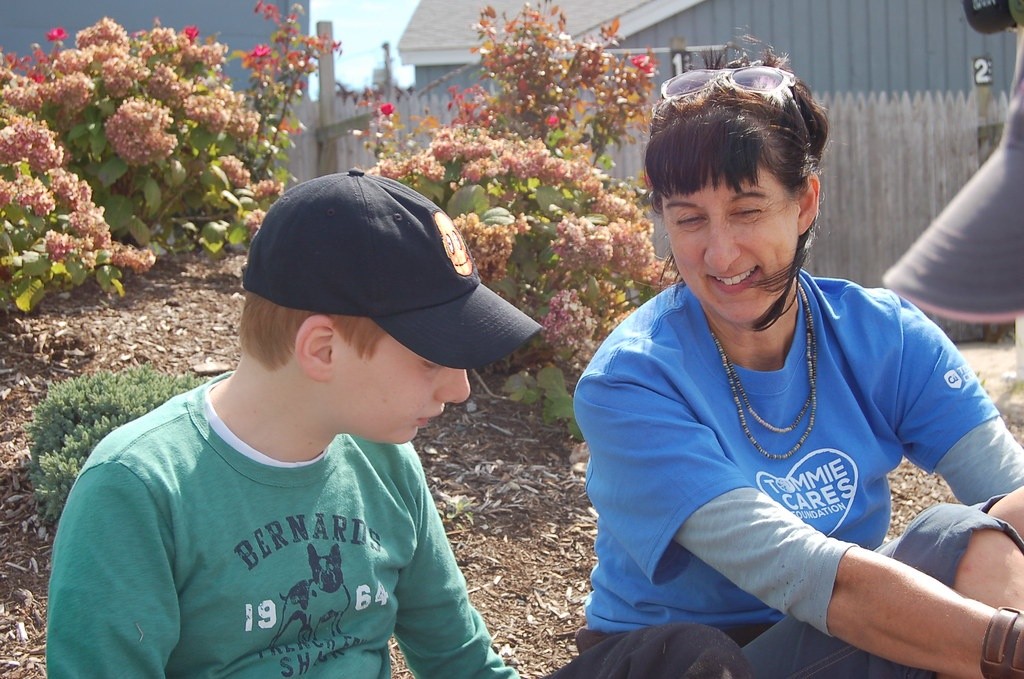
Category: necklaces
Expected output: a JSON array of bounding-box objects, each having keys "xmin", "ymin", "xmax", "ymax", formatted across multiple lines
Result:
[{"xmin": 712, "ymin": 282, "xmax": 817, "ymax": 459}]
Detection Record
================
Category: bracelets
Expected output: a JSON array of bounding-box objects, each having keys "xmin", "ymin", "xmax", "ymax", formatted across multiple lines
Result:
[{"xmin": 980, "ymin": 606, "xmax": 1024, "ymax": 679}]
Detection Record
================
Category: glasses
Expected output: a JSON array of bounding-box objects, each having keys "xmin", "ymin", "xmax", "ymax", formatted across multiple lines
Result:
[{"xmin": 661, "ymin": 65, "xmax": 810, "ymax": 141}]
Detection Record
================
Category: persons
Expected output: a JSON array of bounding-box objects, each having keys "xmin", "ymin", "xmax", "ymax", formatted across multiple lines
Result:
[
  {"xmin": 575, "ymin": 40, "xmax": 1024, "ymax": 678},
  {"xmin": 47, "ymin": 169, "xmax": 546, "ymax": 679}
]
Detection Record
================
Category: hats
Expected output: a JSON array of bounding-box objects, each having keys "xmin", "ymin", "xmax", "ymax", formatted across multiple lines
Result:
[
  {"xmin": 884, "ymin": 53, "xmax": 1024, "ymax": 323},
  {"xmin": 242, "ymin": 168, "xmax": 544, "ymax": 370}
]
